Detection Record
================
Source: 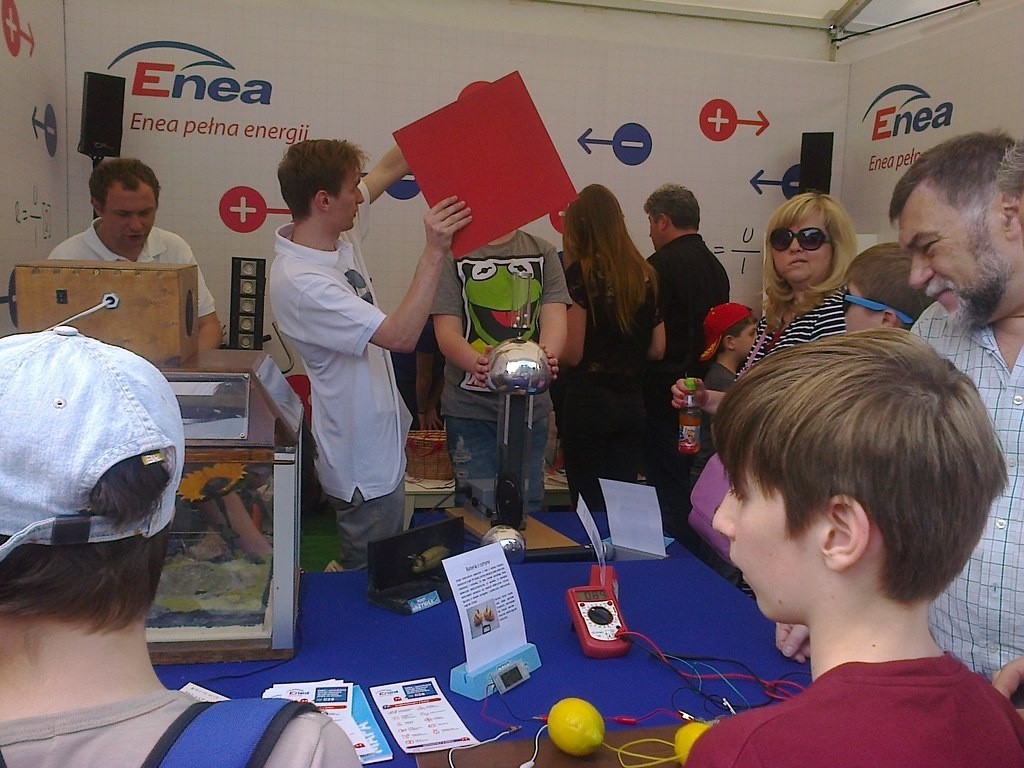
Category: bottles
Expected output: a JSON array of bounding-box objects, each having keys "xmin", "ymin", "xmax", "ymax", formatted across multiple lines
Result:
[{"xmin": 679, "ymin": 379, "xmax": 701, "ymax": 453}]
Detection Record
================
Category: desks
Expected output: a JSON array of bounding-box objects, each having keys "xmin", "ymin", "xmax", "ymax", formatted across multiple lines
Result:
[
  {"xmin": 160, "ymin": 556, "xmax": 811, "ymax": 768},
  {"xmin": 405, "ymin": 508, "xmax": 689, "ymax": 556},
  {"xmin": 404, "ymin": 469, "xmax": 573, "ymax": 540}
]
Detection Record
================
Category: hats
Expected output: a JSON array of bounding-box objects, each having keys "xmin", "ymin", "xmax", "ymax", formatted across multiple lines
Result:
[
  {"xmin": 0, "ymin": 325, "xmax": 186, "ymax": 561},
  {"xmin": 699, "ymin": 303, "xmax": 752, "ymax": 362}
]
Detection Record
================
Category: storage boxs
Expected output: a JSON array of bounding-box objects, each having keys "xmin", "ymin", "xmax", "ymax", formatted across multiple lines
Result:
[{"xmin": 145, "ymin": 395, "xmax": 307, "ymax": 667}]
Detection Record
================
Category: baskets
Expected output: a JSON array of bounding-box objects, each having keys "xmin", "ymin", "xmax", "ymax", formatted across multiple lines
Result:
[{"xmin": 405, "ymin": 429, "xmax": 454, "ymax": 481}]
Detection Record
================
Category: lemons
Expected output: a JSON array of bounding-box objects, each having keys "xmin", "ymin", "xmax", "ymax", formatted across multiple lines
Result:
[
  {"xmin": 548, "ymin": 697, "xmax": 605, "ymax": 756},
  {"xmin": 674, "ymin": 721, "xmax": 712, "ymax": 765}
]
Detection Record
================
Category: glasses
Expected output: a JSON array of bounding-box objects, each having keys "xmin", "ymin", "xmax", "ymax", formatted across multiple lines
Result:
[
  {"xmin": 344, "ymin": 269, "xmax": 374, "ymax": 305},
  {"xmin": 842, "ymin": 289, "xmax": 914, "ymax": 324},
  {"xmin": 770, "ymin": 228, "xmax": 830, "ymax": 251}
]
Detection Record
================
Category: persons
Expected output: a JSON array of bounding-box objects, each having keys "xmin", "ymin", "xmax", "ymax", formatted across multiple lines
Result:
[
  {"xmin": 429, "ymin": 185, "xmax": 730, "ymax": 556},
  {"xmin": 0, "ymin": 326, "xmax": 365, "ymax": 768},
  {"xmin": 684, "ymin": 328, "xmax": 1024, "ymax": 768},
  {"xmin": 672, "ymin": 131, "xmax": 1024, "ymax": 718},
  {"xmin": 270, "ymin": 138, "xmax": 472, "ymax": 572},
  {"xmin": 47, "ymin": 159, "xmax": 222, "ymax": 350}
]
